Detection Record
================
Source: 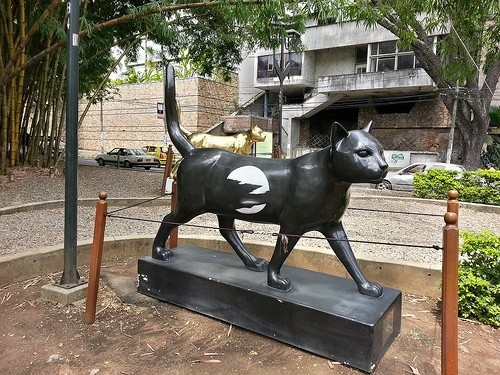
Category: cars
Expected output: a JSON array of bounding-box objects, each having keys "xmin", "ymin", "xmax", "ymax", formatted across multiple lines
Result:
[
  {"xmin": 95, "ymin": 148, "xmax": 160, "ymax": 170},
  {"xmin": 142, "ymin": 145, "xmax": 177, "ymax": 166},
  {"xmin": 369, "ymin": 163, "xmax": 467, "ymax": 192}
]
